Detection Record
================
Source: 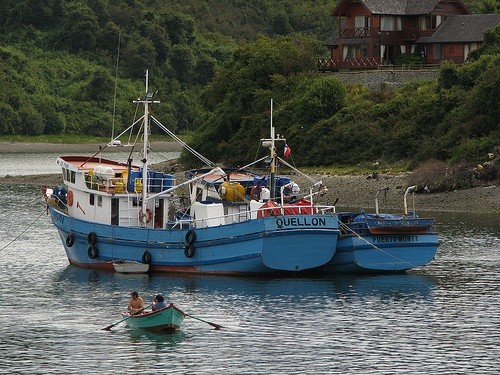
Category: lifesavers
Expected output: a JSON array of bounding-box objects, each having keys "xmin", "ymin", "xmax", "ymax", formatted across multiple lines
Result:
[
  {"xmin": 139, "ymin": 209, "xmax": 152, "ymax": 223},
  {"xmin": 66, "ymin": 234, "xmax": 74, "ymax": 247},
  {"xmin": 87, "ymin": 246, "xmax": 98, "ymax": 259},
  {"xmin": 250, "ymin": 186, "xmax": 262, "ymax": 202},
  {"xmin": 67, "ymin": 190, "xmax": 73, "ymax": 206},
  {"xmin": 184, "ymin": 246, "xmax": 195, "ymax": 258},
  {"xmin": 142, "ymin": 251, "xmax": 151, "ymax": 264},
  {"xmin": 281, "ymin": 185, "xmax": 295, "ymax": 200},
  {"xmin": 87, "ymin": 232, "xmax": 97, "ymax": 245},
  {"xmin": 185, "ymin": 230, "xmax": 196, "ymax": 245}
]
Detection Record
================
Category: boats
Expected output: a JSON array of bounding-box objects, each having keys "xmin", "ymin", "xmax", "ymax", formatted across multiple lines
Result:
[
  {"xmin": 40, "ymin": 66, "xmax": 340, "ymax": 280},
  {"xmin": 120, "ymin": 303, "xmax": 186, "ymax": 332},
  {"xmin": 314, "ymin": 181, "xmax": 441, "ymax": 276}
]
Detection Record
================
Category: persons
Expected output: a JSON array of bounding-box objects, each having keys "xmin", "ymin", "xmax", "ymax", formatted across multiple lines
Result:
[
  {"xmin": 127, "ymin": 291, "xmax": 144, "ymax": 316},
  {"xmin": 152, "ymin": 294, "xmax": 167, "ymax": 311}
]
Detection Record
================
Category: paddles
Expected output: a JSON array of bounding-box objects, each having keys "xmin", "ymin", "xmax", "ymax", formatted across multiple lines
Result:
[
  {"xmin": 185, "ymin": 315, "xmax": 225, "ymax": 329},
  {"xmin": 104, "ymin": 303, "xmax": 153, "ymax": 330}
]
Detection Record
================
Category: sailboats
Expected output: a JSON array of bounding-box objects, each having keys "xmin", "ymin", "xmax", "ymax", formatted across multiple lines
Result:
[{"xmin": 107, "ymin": 29, "xmax": 122, "ymax": 146}]
